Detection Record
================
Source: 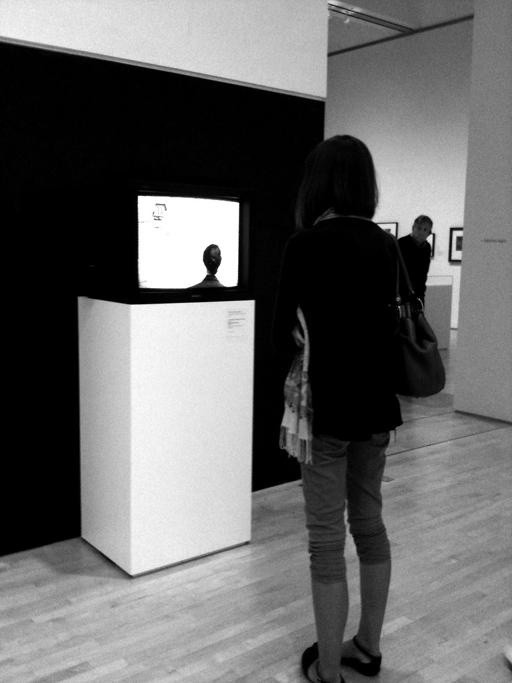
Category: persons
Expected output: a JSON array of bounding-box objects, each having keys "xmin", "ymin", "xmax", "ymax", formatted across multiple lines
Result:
[
  {"xmin": 283, "ymin": 132, "xmax": 401, "ymax": 683},
  {"xmin": 188, "ymin": 243, "xmax": 226, "ymax": 287},
  {"xmin": 397, "ymin": 216, "xmax": 436, "ymax": 311}
]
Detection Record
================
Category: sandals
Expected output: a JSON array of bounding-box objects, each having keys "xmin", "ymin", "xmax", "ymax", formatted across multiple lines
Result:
[
  {"xmin": 313, "ymin": 635, "xmax": 381, "ymax": 676},
  {"xmin": 302, "ymin": 647, "xmax": 345, "ymax": 683}
]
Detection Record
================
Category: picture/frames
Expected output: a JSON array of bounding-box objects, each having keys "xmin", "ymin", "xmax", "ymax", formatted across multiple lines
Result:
[
  {"xmin": 449, "ymin": 223, "xmax": 462, "ymax": 266},
  {"xmin": 426, "ymin": 233, "xmax": 436, "ymax": 258},
  {"xmin": 373, "ymin": 222, "xmax": 398, "ymax": 235}
]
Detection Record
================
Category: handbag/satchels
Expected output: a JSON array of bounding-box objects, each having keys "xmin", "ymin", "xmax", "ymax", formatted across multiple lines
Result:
[{"xmin": 385, "ymin": 233, "xmax": 445, "ymax": 397}]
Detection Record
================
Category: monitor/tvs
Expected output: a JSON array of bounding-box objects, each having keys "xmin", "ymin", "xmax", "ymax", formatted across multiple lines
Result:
[{"xmin": 131, "ymin": 189, "xmax": 249, "ymax": 293}]
